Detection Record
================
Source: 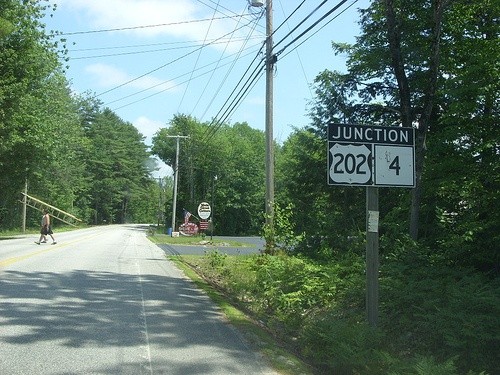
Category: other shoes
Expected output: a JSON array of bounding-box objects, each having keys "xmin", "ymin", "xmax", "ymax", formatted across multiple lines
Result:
[
  {"xmin": 51, "ymin": 242, "xmax": 57, "ymax": 245},
  {"xmin": 34, "ymin": 241, "xmax": 40, "ymax": 245},
  {"xmin": 41, "ymin": 239, "xmax": 48, "ymax": 243}
]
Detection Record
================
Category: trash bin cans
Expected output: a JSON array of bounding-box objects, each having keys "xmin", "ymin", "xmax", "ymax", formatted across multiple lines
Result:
[{"xmin": 168, "ymin": 227, "xmax": 172, "ymax": 236}]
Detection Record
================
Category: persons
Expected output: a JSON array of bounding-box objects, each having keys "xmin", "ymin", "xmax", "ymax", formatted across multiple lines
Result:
[
  {"xmin": 40, "ymin": 209, "xmax": 49, "ymax": 243},
  {"xmin": 34, "ymin": 209, "xmax": 56, "ymax": 245}
]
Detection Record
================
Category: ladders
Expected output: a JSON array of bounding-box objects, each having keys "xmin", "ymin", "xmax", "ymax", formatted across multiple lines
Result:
[{"xmin": 18, "ymin": 192, "xmax": 83, "ymax": 228}]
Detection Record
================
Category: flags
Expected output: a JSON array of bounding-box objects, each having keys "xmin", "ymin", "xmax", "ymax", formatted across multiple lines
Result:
[{"xmin": 183, "ymin": 209, "xmax": 191, "ymax": 227}]
